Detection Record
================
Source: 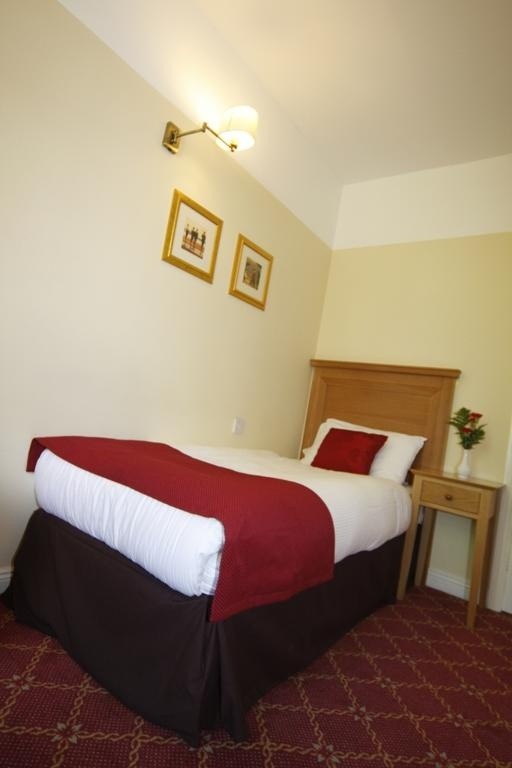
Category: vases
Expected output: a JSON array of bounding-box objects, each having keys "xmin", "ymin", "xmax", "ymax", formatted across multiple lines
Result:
[{"xmin": 456, "ymin": 440, "xmax": 472, "ymax": 479}]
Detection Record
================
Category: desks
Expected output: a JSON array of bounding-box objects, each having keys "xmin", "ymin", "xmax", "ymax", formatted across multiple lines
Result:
[{"xmin": 394, "ymin": 468, "xmax": 508, "ymax": 635}]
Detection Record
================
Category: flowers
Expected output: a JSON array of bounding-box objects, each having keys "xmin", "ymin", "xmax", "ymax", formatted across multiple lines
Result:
[{"xmin": 443, "ymin": 401, "xmax": 489, "ymax": 451}]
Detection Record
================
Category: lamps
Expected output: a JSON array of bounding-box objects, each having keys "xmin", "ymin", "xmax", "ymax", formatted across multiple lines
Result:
[{"xmin": 162, "ymin": 113, "xmax": 254, "ymax": 157}]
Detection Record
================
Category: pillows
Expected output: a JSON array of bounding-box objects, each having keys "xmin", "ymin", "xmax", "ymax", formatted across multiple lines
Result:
[
  {"xmin": 300, "ymin": 422, "xmax": 429, "ymax": 487},
  {"xmin": 309, "ymin": 426, "xmax": 388, "ymax": 477}
]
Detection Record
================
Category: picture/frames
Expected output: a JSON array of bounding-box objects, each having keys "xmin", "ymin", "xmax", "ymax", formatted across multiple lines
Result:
[
  {"xmin": 228, "ymin": 230, "xmax": 275, "ymax": 313},
  {"xmin": 160, "ymin": 186, "xmax": 225, "ymax": 286}
]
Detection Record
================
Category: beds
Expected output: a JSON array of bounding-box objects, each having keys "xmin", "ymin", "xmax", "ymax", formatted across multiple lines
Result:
[{"xmin": 1, "ymin": 357, "xmax": 464, "ymax": 751}]
